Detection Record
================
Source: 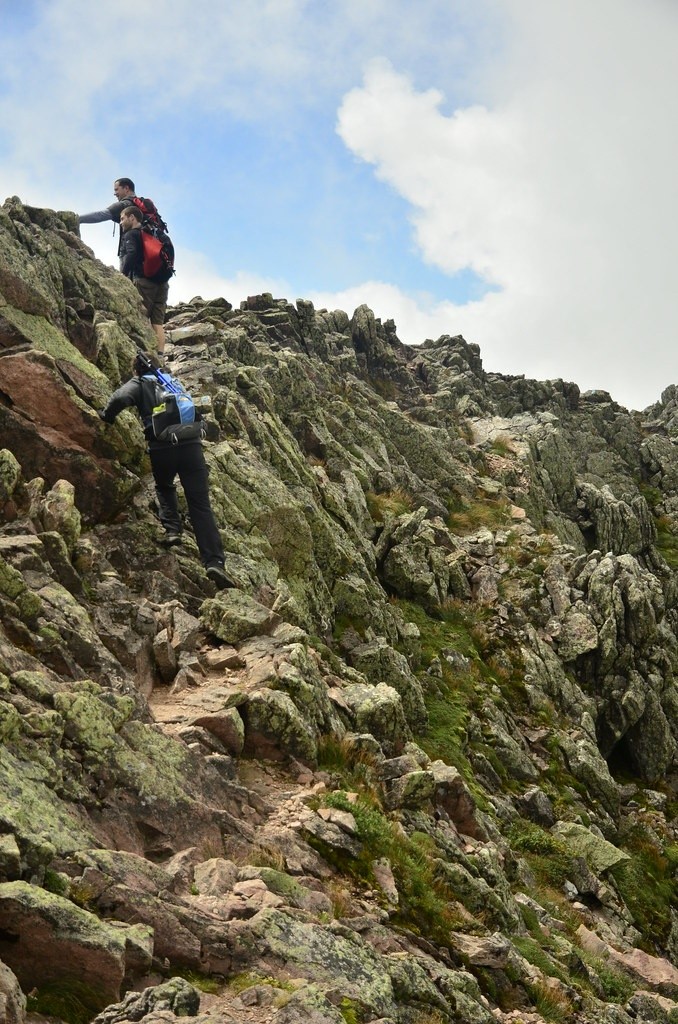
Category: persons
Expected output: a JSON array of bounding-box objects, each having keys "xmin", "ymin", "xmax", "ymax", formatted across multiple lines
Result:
[
  {"xmin": 119, "ymin": 206, "xmax": 170, "ymax": 358},
  {"xmin": 80, "ymin": 178, "xmax": 143, "ymax": 281},
  {"xmin": 97, "ymin": 353, "xmax": 236, "ymax": 590}
]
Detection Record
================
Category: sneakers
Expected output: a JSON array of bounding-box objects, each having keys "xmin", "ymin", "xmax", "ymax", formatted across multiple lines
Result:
[
  {"xmin": 205, "ymin": 567, "xmax": 235, "ymax": 590},
  {"xmin": 156, "ymin": 531, "xmax": 184, "ymax": 547}
]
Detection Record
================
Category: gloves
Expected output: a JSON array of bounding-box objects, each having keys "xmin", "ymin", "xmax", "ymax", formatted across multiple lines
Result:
[{"xmin": 98, "ymin": 410, "xmax": 115, "ymax": 424}]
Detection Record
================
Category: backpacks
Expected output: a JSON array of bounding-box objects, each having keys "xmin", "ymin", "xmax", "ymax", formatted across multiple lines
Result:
[
  {"xmin": 134, "ymin": 226, "xmax": 175, "ymax": 281},
  {"xmin": 121, "ymin": 196, "xmax": 168, "ymax": 233},
  {"xmin": 143, "ymin": 367, "xmax": 208, "ymax": 445}
]
